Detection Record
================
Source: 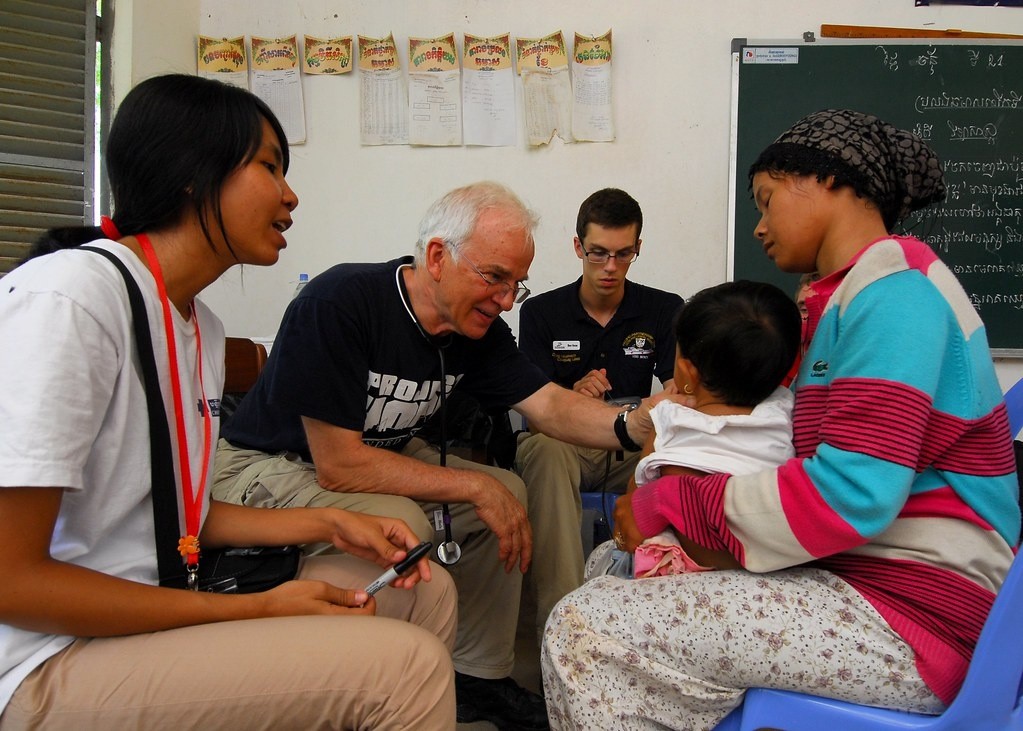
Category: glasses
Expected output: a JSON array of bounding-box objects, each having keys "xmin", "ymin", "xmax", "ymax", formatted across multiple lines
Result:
[
  {"xmin": 580, "ymin": 241, "xmax": 639, "ymax": 264},
  {"xmin": 444, "ymin": 240, "xmax": 531, "ymax": 303}
]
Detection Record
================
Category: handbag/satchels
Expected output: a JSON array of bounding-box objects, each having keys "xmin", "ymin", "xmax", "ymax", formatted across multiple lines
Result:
[{"xmin": 171, "ymin": 545, "xmax": 302, "ymax": 594}]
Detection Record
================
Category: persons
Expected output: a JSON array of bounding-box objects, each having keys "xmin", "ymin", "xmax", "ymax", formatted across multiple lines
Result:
[
  {"xmin": 540, "ymin": 109, "xmax": 1023, "ymax": 729},
  {"xmin": 210, "ymin": 176, "xmax": 698, "ymax": 731},
  {"xmin": 582, "ymin": 277, "xmax": 803, "ymax": 586},
  {"xmin": 515, "ymin": 184, "xmax": 687, "ymax": 651},
  {"xmin": 0, "ymin": 75, "xmax": 460, "ymax": 731}
]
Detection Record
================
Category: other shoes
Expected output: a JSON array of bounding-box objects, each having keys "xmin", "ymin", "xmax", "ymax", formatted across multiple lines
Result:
[{"xmin": 454, "ymin": 672, "xmax": 550, "ymax": 731}]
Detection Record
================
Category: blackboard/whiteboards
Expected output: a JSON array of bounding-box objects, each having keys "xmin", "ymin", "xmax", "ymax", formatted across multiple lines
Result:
[{"xmin": 728, "ymin": 34, "xmax": 1023, "ymax": 364}]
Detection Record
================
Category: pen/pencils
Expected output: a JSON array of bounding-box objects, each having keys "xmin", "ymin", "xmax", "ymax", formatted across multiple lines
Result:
[{"xmin": 368, "ymin": 539, "xmax": 433, "ymax": 596}]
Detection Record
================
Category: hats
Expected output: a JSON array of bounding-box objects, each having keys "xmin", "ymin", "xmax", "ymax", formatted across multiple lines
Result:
[{"xmin": 748, "ymin": 109, "xmax": 946, "ymax": 231}]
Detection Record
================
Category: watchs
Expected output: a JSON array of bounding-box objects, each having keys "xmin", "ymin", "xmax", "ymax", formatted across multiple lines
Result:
[{"xmin": 612, "ymin": 403, "xmax": 643, "ymax": 452}]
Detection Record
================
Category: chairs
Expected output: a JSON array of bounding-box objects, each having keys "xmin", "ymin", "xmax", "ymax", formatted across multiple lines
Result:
[{"xmin": 713, "ymin": 377, "xmax": 1023, "ymax": 731}]
